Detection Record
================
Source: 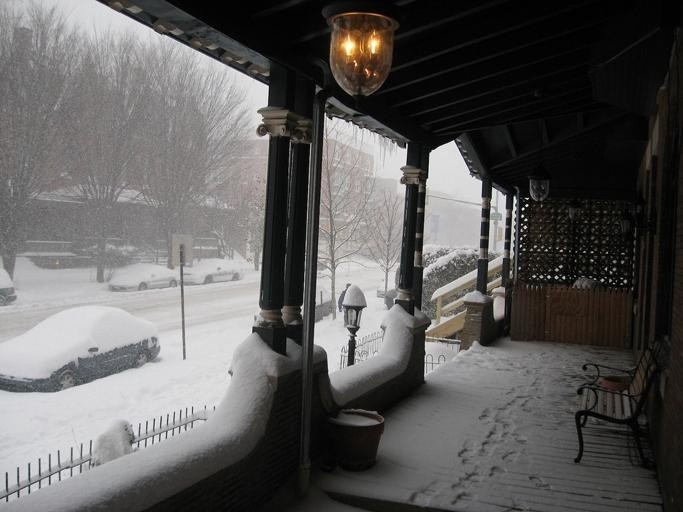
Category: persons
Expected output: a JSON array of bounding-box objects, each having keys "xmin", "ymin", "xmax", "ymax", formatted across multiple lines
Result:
[{"xmin": 337, "ymin": 282, "xmax": 351, "ymax": 312}]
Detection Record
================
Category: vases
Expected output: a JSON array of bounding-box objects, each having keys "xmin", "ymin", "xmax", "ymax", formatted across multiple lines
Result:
[{"xmin": 328, "ymin": 409, "xmax": 385, "ymax": 470}]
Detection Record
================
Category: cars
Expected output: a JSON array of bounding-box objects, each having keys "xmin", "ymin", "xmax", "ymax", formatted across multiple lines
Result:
[
  {"xmin": 108, "ymin": 264, "xmax": 178, "ymax": 292},
  {"xmin": 181, "ymin": 259, "xmax": 243, "ymax": 286},
  {"xmin": 0, "ymin": 306, "xmax": 161, "ymax": 393}
]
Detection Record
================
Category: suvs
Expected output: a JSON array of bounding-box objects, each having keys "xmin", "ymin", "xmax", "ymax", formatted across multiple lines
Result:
[{"xmin": 0, "ymin": 269, "xmax": 17, "ymax": 306}]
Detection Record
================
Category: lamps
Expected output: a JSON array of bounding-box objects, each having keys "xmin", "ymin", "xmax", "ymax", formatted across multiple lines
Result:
[
  {"xmin": 320, "ymin": 1, "xmax": 401, "ymax": 114},
  {"xmin": 528, "ymin": 170, "xmax": 553, "ymax": 201}
]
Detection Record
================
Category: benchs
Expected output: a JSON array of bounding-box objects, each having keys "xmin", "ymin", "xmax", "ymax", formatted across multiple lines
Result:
[{"xmin": 572, "ymin": 339, "xmax": 661, "ymax": 467}]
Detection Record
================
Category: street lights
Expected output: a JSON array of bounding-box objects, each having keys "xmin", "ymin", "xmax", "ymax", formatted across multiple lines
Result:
[{"xmin": 342, "ymin": 285, "xmax": 366, "ymax": 369}]
